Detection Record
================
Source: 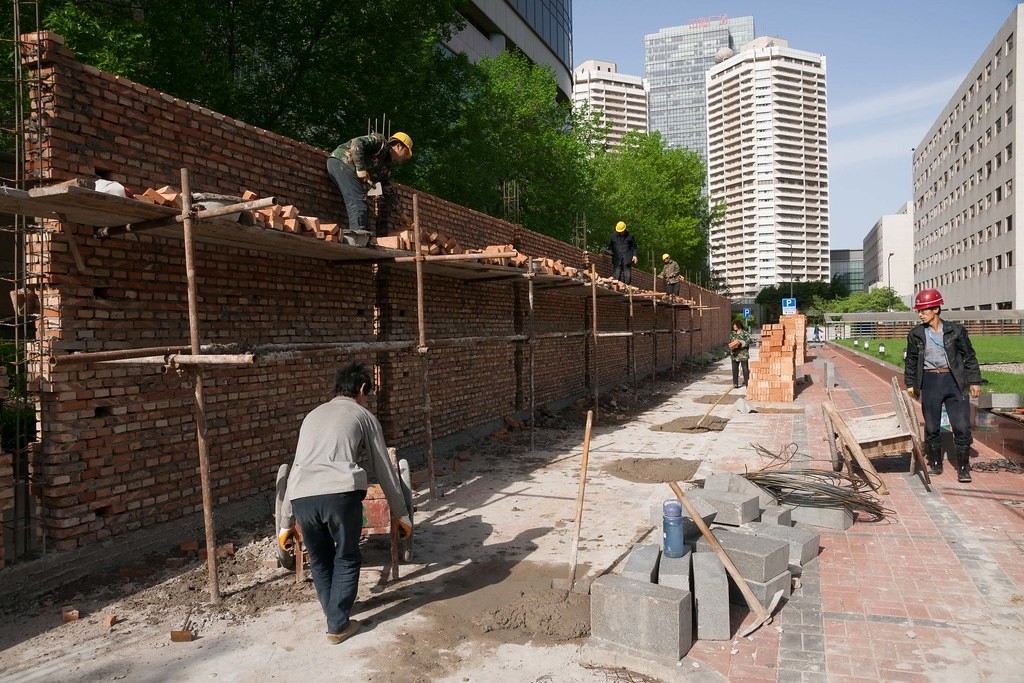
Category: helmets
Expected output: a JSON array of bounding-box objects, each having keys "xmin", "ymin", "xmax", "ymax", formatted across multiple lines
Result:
[
  {"xmin": 663, "ymin": 253, "xmax": 670, "ymax": 260},
  {"xmin": 616, "ymin": 221, "xmax": 627, "ymax": 232},
  {"xmin": 913, "ymin": 289, "xmax": 944, "ymax": 310},
  {"xmin": 388, "ymin": 132, "xmax": 413, "ymax": 160}
]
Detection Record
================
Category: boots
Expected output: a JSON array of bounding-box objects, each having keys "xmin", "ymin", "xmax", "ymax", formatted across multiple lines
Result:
[
  {"xmin": 957, "ymin": 446, "xmax": 972, "ymax": 481},
  {"xmin": 930, "ymin": 445, "xmax": 942, "ymax": 474}
]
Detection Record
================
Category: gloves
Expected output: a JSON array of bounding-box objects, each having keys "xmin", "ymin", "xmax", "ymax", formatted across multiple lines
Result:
[
  {"xmin": 392, "ymin": 513, "xmax": 412, "ymax": 540},
  {"xmin": 279, "ymin": 527, "xmax": 294, "ymax": 549},
  {"xmin": 907, "ymin": 387, "xmax": 914, "ymax": 397},
  {"xmin": 632, "ymin": 257, "xmax": 638, "ymax": 264}
]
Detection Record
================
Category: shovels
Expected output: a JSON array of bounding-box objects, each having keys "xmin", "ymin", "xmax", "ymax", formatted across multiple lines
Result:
[
  {"xmin": 682, "ymin": 386, "xmax": 734, "ymax": 429},
  {"xmin": 551, "ymin": 410, "xmax": 593, "ymax": 595}
]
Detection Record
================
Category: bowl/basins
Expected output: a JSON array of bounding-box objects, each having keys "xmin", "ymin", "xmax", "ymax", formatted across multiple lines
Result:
[{"xmin": 191, "ymin": 193, "xmax": 243, "ymax": 223}]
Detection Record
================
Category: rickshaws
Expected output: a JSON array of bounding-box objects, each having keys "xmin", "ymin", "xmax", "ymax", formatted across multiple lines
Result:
[
  {"xmin": 274, "ymin": 445, "xmax": 414, "ymax": 585},
  {"xmin": 821, "ymin": 376, "xmax": 932, "ymax": 494}
]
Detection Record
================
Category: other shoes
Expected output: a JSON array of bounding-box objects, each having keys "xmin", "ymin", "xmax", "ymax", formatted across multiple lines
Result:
[{"xmin": 733, "ymin": 384, "xmax": 738, "ymax": 388}]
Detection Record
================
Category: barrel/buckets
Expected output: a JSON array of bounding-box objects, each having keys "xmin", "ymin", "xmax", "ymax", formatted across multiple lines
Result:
[
  {"xmin": 576, "ymin": 269, "xmax": 584, "ymax": 278},
  {"xmin": 603, "ymin": 280, "xmax": 613, "ymax": 288},
  {"xmin": 939, "ymin": 407, "xmax": 950, "ymax": 431},
  {"xmin": 343, "ymin": 230, "xmax": 373, "ymax": 248},
  {"xmin": 526, "ymin": 260, "xmax": 543, "ymax": 268}
]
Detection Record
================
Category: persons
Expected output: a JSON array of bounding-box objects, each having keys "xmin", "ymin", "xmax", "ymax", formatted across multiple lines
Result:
[
  {"xmin": 606, "ymin": 221, "xmax": 639, "ymax": 285},
  {"xmin": 835, "ymin": 326, "xmax": 840, "ymax": 340},
  {"xmin": 659, "ymin": 254, "xmax": 681, "ymax": 296},
  {"xmin": 904, "ymin": 288, "xmax": 981, "ymax": 481},
  {"xmin": 326, "ymin": 132, "xmax": 413, "ymax": 230},
  {"xmin": 728, "ymin": 319, "xmax": 751, "ymax": 388},
  {"xmin": 814, "ymin": 324, "xmax": 823, "ymax": 341},
  {"xmin": 279, "ymin": 360, "xmax": 412, "ymax": 645}
]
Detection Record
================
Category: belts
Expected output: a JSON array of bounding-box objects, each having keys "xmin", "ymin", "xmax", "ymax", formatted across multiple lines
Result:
[{"xmin": 925, "ymin": 368, "xmax": 950, "ymax": 373}]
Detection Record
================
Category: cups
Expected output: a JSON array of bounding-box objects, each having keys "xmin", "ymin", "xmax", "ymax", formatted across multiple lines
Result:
[{"xmin": 662, "ymin": 499, "xmax": 684, "ymax": 558}]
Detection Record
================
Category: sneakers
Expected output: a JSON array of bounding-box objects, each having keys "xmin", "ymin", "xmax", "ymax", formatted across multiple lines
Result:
[{"xmin": 328, "ymin": 620, "xmax": 360, "ymax": 644}]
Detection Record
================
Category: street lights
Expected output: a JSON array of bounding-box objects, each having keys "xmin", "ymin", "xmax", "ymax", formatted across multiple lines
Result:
[
  {"xmin": 788, "ymin": 243, "xmax": 793, "ymax": 298},
  {"xmin": 887, "ymin": 253, "xmax": 894, "ymax": 313}
]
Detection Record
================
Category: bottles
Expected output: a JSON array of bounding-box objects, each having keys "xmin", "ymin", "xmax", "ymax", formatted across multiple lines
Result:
[{"xmin": 986, "ymin": 388, "xmax": 995, "ymax": 393}]
